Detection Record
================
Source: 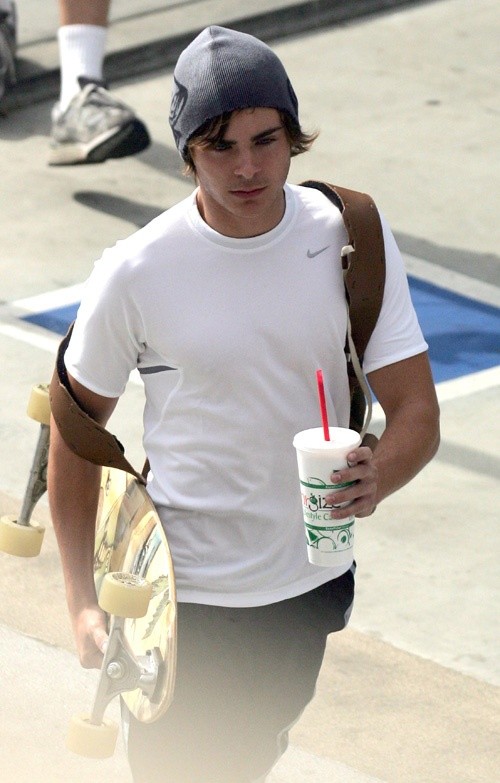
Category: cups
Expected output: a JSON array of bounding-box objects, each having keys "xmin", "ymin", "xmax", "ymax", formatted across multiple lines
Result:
[{"xmin": 292, "ymin": 427, "xmax": 362, "ymax": 568}]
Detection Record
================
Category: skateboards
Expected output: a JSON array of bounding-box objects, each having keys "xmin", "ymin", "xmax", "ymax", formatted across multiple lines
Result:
[{"xmin": 2, "ymin": 384, "xmax": 177, "ymax": 759}]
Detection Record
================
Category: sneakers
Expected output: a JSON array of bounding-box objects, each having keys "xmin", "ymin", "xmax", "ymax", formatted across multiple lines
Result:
[
  {"xmin": 0, "ymin": 10, "xmax": 17, "ymax": 97},
  {"xmin": 46, "ymin": 76, "xmax": 151, "ymax": 166}
]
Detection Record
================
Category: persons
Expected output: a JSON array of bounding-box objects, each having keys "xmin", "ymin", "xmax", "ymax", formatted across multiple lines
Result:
[
  {"xmin": 47, "ymin": 25, "xmax": 440, "ymax": 783},
  {"xmin": 48, "ymin": 0, "xmax": 152, "ymax": 166}
]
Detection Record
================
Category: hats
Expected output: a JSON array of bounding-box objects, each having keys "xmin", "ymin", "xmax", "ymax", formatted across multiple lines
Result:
[{"xmin": 169, "ymin": 25, "xmax": 299, "ymax": 164}]
